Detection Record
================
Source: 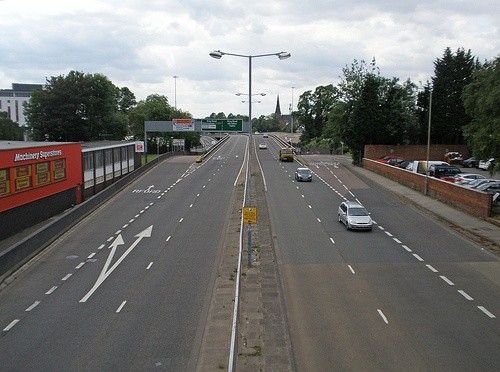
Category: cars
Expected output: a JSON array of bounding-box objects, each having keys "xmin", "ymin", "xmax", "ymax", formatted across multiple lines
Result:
[
  {"xmin": 263, "ymin": 134, "xmax": 269, "ymax": 138},
  {"xmin": 376, "ymin": 152, "xmax": 500, "ymax": 199},
  {"xmin": 294, "ymin": 168, "xmax": 313, "ymax": 181},
  {"xmin": 259, "ymin": 143, "xmax": 267, "ymax": 149},
  {"xmin": 254, "ymin": 131, "xmax": 259, "ymax": 135},
  {"xmin": 337, "ymin": 202, "xmax": 373, "ymax": 231}
]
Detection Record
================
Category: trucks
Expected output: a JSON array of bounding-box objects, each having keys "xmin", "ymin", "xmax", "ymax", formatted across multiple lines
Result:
[{"xmin": 279, "ymin": 147, "xmax": 294, "ymax": 162}]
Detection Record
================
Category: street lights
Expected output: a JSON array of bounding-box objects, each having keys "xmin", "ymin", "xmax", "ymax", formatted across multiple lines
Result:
[
  {"xmin": 209, "ymin": 48, "xmax": 291, "ymax": 270},
  {"xmin": 172, "ymin": 75, "xmax": 178, "ymax": 109}
]
